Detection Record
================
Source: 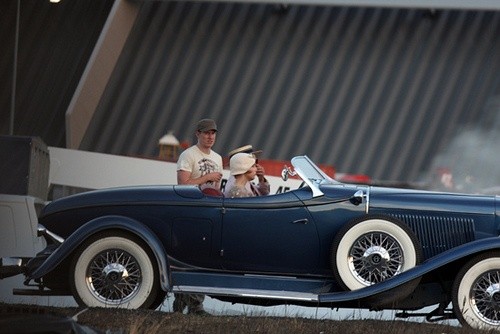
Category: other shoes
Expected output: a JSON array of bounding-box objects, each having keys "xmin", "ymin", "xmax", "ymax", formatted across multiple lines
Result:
[{"xmin": 173, "ymin": 310, "xmax": 212, "ymax": 316}]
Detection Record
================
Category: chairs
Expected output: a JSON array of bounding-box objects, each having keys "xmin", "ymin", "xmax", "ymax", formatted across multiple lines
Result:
[{"xmin": 202, "ymin": 187, "xmax": 225, "ymax": 199}]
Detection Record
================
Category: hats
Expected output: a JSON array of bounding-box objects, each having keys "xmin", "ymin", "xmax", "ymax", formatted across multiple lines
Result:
[
  {"xmin": 196, "ymin": 119, "xmax": 218, "ymax": 132},
  {"xmin": 227, "ymin": 145, "xmax": 263, "ymax": 161},
  {"xmin": 230, "ymin": 153, "xmax": 258, "ymax": 176}
]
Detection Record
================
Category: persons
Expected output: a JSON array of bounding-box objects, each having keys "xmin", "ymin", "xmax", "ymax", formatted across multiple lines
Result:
[
  {"xmin": 230, "ymin": 152, "xmax": 258, "ymax": 199},
  {"xmin": 172, "ymin": 119, "xmax": 223, "ymax": 316},
  {"xmin": 224, "ymin": 144, "xmax": 270, "ymax": 200}
]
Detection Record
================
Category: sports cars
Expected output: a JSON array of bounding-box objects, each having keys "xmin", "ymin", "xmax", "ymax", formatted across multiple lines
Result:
[{"xmin": 12, "ymin": 154, "xmax": 500, "ymax": 334}]
